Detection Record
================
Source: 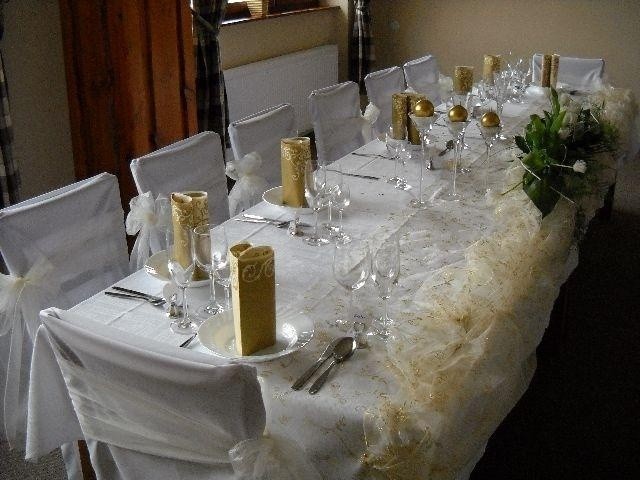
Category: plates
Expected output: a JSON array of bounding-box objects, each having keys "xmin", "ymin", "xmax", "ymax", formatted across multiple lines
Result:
[
  {"xmin": 530, "ymin": 82, "xmax": 566, "ymax": 91},
  {"xmin": 262, "ymin": 186, "xmax": 329, "ymax": 214},
  {"xmin": 196, "ymin": 309, "xmax": 313, "ymax": 362},
  {"xmin": 143, "ymin": 250, "xmax": 213, "ymax": 288}
]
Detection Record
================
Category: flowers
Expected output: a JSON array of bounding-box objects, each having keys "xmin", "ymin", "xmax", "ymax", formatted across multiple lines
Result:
[{"xmin": 475, "ymin": 89, "xmax": 626, "ymax": 227}]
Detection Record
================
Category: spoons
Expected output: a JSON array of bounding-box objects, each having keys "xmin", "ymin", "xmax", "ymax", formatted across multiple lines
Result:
[
  {"xmin": 103, "ymin": 290, "xmax": 165, "ymax": 306},
  {"xmin": 308, "ymin": 337, "xmax": 357, "ymax": 394},
  {"xmin": 234, "ymin": 215, "xmax": 293, "ymax": 228},
  {"xmin": 352, "ymin": 152, "xmax": 399, "ymax": 160}
]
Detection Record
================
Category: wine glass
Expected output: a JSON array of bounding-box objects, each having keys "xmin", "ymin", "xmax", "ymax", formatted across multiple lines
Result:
[
  {"xmin": 161, "ymin": 223, "xmax": 233, "ymax": 337},
  {"xmin": 384, "ymin": 60, "xmax": 535, "ymax": 209},
  {"xmin": 332, "ymin": 242, "xmax": 401, "ymax": 350},
  {"xmin": 303, "ymin": 159, "xmax": 352, "ymax": 247}
]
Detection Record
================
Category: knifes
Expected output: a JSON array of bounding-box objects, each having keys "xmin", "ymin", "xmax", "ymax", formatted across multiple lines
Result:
[
  {"xmin": 242, "ymin": 212, "xmax": 312, "ymax": 229},
  {"xmin": 328, "ymin": 168, "xmax": 379, "ymax": 180},
  {"xmin": 113, "ymin": 286, "xmax": 163, "ymax": 300},
  {"xmin": 291, "ymin": 336, "xmax": 343, "ymax": 391}
]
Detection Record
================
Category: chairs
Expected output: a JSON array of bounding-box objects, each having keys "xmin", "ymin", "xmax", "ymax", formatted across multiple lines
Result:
[
  {"xmin": 1, "ymin": 170, "xmax": 134, "ymax": 352},
  {"xmin": 402, "ymin": 54, "xmax": 444, "ymax": 112},
  {"xmin": 304, "ymin": 78, "xmax": 367, "ymax": 173},
  {"xmin": 228, "ymin": 102, "xmax": 305, "ymax": 214},
  {"xmin": 30, "ymin": 303, "xmax": 273, "ymax": 480},
  {"xmin": 362, "ymin": 64, "xmax": 410, "ymax": 140},
  {"xmin": 125, "ymin": 130, "xmax": 233, "ymax": 258},
  {"xmin": 530, "ymin": 52, "xmax": 605, "ymax": 98}
]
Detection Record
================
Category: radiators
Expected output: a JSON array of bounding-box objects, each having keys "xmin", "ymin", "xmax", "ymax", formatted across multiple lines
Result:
[{"xmin": 220, "ymin": 42, "xmax": 340, "ymax": 146}]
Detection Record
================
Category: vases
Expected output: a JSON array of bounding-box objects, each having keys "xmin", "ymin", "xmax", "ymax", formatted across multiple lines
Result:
[{"xmin": 245, "ymin": 1, "xmax": 269, "ymax": 18}]
